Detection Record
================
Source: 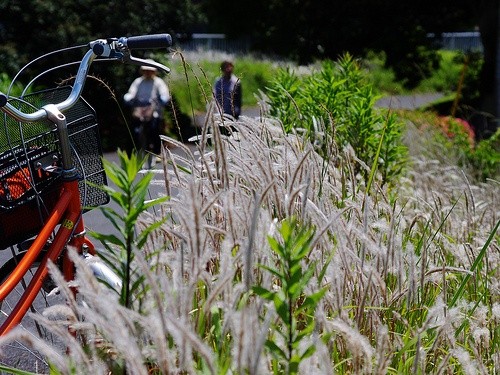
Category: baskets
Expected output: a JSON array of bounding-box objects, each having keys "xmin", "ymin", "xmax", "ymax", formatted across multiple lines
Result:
[{"xmin": 0, "ymin": 85, "xmax": 110, "ymax": 249}]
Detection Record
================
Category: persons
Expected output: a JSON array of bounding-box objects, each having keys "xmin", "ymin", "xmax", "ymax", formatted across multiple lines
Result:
[
  {"xmin": 213, "ymin": 61, "xmax": 243, "ymax": 137},
  {"xmin": 123, "ymin": 58, "xmax": 171, "ymax": 151}
]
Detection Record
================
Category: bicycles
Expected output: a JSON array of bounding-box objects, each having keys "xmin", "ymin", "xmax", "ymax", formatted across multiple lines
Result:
[
  {"xmin": 129, "ymin": 98, "xmax": 163, "ymax": 168},
  {"xmin": 0, "ymin": 33, "xmax": 173, "ymax": 345}
]
